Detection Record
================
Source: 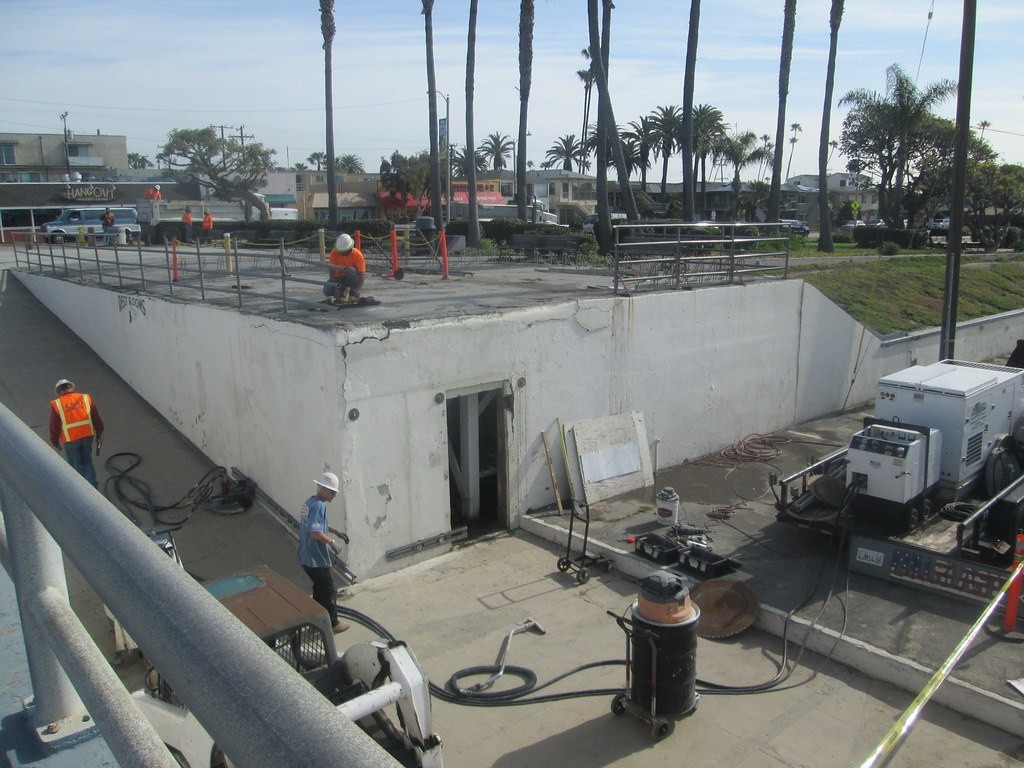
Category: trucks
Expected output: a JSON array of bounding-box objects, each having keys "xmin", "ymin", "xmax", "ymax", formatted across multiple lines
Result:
[{"xmin": 582, "ymin": 213, "xmax": 641, "ymax": 234}]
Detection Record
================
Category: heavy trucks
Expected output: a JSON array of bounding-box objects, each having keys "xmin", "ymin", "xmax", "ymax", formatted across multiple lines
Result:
[
  {"xmin": 135, "ymin": 192, "xmax": 298, "ymax": 246},
  {"xmin": 447, "ymin": 196, "xmax": 558, "ymax": 225}
]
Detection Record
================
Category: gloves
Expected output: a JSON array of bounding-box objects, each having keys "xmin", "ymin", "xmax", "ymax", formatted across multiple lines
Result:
[
  {"xmin": 336, "ymin": 529, "xmax": 349, "ymax": 544},
  {"xmin": 330, "ymin": 540, "xmax": 341, "ymax": 555}
]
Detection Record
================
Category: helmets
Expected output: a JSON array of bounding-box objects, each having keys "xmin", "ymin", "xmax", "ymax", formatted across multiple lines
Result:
[
  {"xmin": 55, "ymin": 379, "xmax": 75, "ymax": 393},
  {"xmin": 334, "ymin": 234, "xmax": 354, "ymax": 252},
  {"xmin": 312, "ymin": 473, "xmax": 339, "ymax": 492},
  {"xmin": 155, "ymin": 185, "xmax": 160, "ymax": 190}
]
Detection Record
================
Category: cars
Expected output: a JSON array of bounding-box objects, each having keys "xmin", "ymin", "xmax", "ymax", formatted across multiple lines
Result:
[
  {"xmin": 867, "ymin": 219, "xmax": 888, "ymax": 228},
  {"xmin": 841, "ymin": 220, "xmax": 866, "ymax": 229}
]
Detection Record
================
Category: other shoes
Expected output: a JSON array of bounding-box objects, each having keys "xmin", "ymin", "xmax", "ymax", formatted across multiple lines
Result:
[{"xmin": 333, "ymin": 623, "xmax": 349, "ymax": 633}]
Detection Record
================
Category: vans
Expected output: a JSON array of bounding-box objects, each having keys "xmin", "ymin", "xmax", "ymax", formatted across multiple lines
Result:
[
  {"xmin": 40, "ymin": 207, "xmax": 142, "ymax": 244},
  {"xmin": 933, "ymin": 218, "xmax": 950, "ymax": 229},
  {"xmin": 778, "ymin": 219, "xmax": 810, "ymax": 236}
]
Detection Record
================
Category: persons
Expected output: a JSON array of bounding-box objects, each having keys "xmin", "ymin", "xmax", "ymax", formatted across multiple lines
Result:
[
  {"xmin": 200, "ymin": 208, "xmax": 213, "ymax": 244},
  {"xmin": 297, "ymin": 472, "xmax": 350, "ymax": 635},
  {"xmin": 182, "ymin": 207, "xmax": 196, "ymax": 244},
  {"xmin": 50, "ymin": 379, "xmax": 104, "ymax": 491},
  {"xmin": 323, "ymin": 233, "xmax": 366, "ymax": 303},
  {"xmin": 145, "ymin": 185, "xmax": 162, "ymax": 202},
  {"xmin": 100, "ymin": 207, "xmax": 115, "ymax": 246}
]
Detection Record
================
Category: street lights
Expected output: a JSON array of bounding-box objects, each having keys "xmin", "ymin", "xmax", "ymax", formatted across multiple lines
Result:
[
  {"xmin": 425, "ymin": 90, "xmax": 450, "ymax": 225},
  {"xmin": 513, "ymin": 131, "xmax": 532, "ymax": 194}
]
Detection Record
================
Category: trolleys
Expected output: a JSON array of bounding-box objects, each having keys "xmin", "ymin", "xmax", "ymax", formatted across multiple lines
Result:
[{"xmin": 556, "ymin": 497, "xmax": 615, "ymax": 583}]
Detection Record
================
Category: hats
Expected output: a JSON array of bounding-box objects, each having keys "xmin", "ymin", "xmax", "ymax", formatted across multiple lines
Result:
[{"xmin": 203, "ymin": 208, "xmax": 210, "ymax": 212}]
